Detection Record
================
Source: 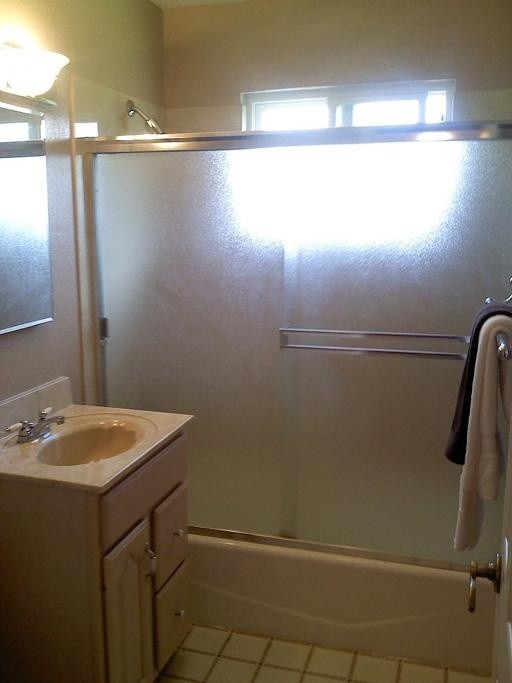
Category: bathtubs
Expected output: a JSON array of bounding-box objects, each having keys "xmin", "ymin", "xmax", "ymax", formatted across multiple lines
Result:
[{"xmin": 186, "ymin": 527, "xmax": 498, "ymax": 676}]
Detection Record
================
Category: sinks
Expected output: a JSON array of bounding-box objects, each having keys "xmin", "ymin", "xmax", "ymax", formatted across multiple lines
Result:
[{"xmin": 35, "ymin": 418, "xmax": 146, "ymax": 467}]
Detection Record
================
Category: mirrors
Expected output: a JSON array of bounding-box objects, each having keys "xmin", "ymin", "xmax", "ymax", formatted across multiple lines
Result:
[{"xmin": 0, "ymin": 99, "xmax": 54, "ymax": 334}]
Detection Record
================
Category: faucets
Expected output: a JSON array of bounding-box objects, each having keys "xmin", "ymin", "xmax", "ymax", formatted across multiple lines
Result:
[{"xmin": 32, "ymin": 416, "xmax": 64, "ymax": 433}]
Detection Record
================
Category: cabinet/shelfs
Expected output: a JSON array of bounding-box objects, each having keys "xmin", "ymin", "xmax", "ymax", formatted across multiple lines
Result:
[{"xmin": 0, "ymin": 431, "xmax": 194, "ymax": 682}]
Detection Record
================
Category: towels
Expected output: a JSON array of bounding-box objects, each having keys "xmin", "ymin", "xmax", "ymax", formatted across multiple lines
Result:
[
  {"xmin": 453, "ymin": 313, "xmax": 512, "ymax": 551},
  {"xmin": 443, "ymin": 304, "xmax": 512, "ymax": 465}
]
